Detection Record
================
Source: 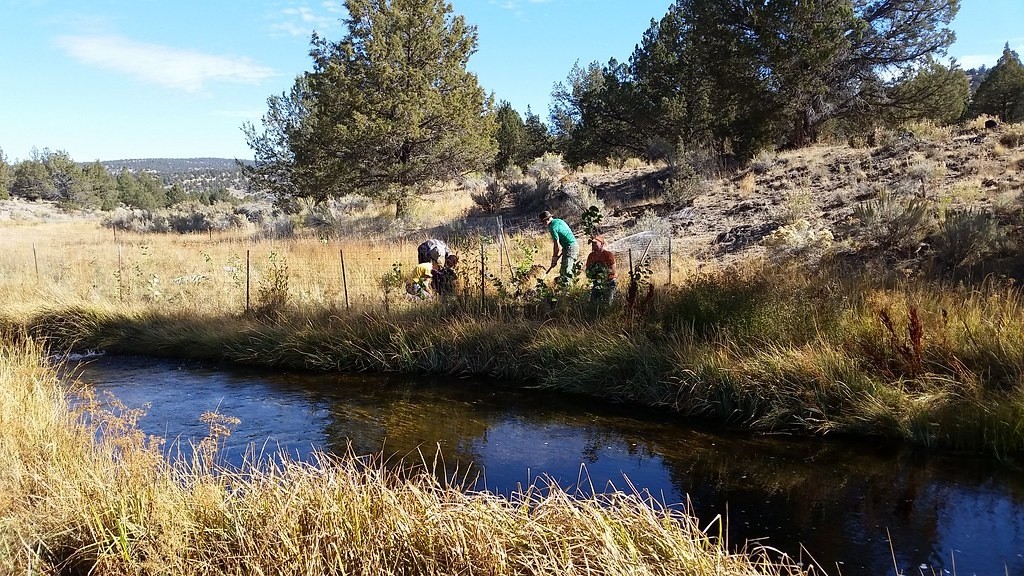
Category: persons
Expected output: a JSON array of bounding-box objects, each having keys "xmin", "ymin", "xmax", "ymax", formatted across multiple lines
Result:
[
  {"xmin": 539, "ymin": 211, "xmax": 579, "ymax": 289},
  {"xmin": 585, "ymin": 236, "xmax": 619, "ymax": 305},
  {"xmin": 405, "ymin": 239, "xmax": 464, "ymax": 303}
]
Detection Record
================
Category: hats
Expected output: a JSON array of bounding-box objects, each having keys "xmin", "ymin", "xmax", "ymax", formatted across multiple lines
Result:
[
  {"xmin": 538, "ymin": 211, "xmax": 555, "ymax": 223},
  {"xmin": 588, "ymin": 235, "xmax": 604, "ymax": 250}
]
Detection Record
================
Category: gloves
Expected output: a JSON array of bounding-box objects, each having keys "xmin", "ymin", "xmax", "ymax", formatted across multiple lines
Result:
[{"xmin": 551, "ymin": 256, "xmax": 559, "ymax": 267}]
